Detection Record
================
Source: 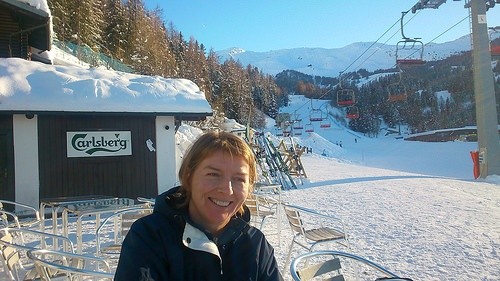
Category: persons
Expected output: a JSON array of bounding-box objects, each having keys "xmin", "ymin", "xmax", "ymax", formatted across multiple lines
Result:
[{"xmin": 114, "ymin": 129, "xmax": 285, "ymax": 281}]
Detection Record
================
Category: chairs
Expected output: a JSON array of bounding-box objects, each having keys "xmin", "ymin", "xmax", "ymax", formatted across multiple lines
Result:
[
  {"xmin": 0, "ymin": 200, "xmax": 47, "ymax": 248},
  {"xmin": 96, "ymin": 208, "xmax": 154, "ymax": 273},
  {"xmin": 138, "ymin": 196, "xmax": 155, "ymax": 208},
  {"xmin": 0, "ymin": 211, "xmax": 27, "ymax": 256},
  {"xmin": 245, "ymin": 195, "xmax": 278, "ymax": 233},
  {"xmin": 291, "ymin": 251, "xmax": 412, "ymax": 281},
  {"xmin": 281, "ymin": 202, "xmax": 361, "ymax": 281},
  {"xmin": 26, "ymin": 248, "xmax": 115, "ymax": 281},
  {"xmin": 0, "ymin": 227, "xmax": 76, "ymax": 281}
]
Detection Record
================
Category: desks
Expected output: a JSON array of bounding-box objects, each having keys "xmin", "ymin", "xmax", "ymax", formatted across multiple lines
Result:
[
  {"xmin": 255, "ymin": 183, "xmax": 282, "ymax": 214},
  {"xmin": 40, "ymin": 195, "xmax": 118, "ymax": 251},
  {"xmin": 62, "ymin": 204, "xmax": 144, "ymax": 255}
]
